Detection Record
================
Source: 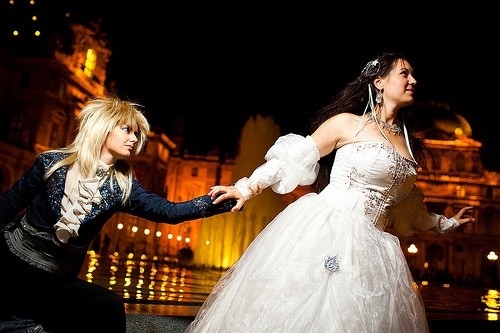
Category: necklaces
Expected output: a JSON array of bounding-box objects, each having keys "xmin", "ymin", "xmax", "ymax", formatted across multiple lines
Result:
[{"xmin": 366, "ymin": 110, "xmax": 402, "ymax": 135}]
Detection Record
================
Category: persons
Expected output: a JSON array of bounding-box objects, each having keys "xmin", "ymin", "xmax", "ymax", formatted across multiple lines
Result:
[
  {"xmin": 0, "ymin": 92, "xmax": 262, "ymax": 333},
  {"xmin": 183, "ymin": 51, "xmax": 477, "ymax": 333}
]
